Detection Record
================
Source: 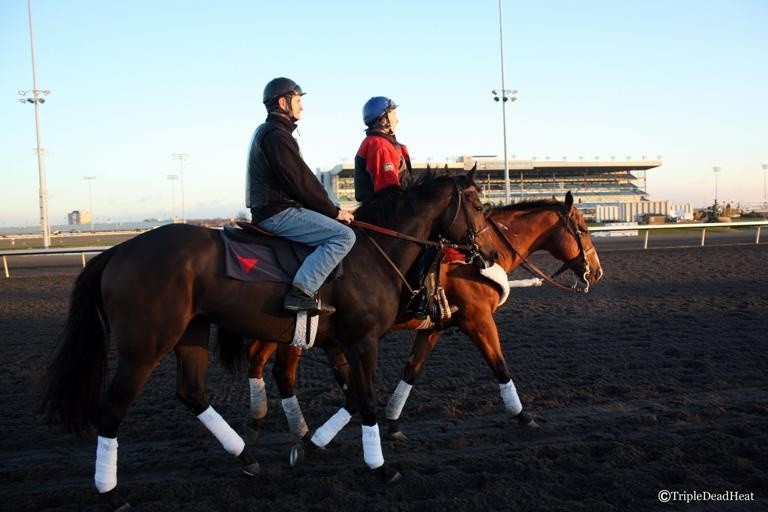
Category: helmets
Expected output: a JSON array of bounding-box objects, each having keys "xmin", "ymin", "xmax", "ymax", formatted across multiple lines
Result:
[
  {"xmin": 363, "ymin": 96, "xmax": 399, "ymax": 125},
  {"xmin": 263, "ymin": 77, "xmax": 307, "ymax": 104}
]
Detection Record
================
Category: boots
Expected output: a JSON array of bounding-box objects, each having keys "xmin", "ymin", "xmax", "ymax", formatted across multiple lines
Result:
[
  {"xmin": 415, "ymin": 250, "xmax": 458, "ymax": 320},
  {"xmin": 284, "ymin": 286, "xmax": 335, "ymax": 316}
]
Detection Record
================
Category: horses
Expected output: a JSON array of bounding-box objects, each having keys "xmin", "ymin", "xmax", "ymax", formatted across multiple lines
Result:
[
  {"xmin": 28, "ymin": 160, "xmax": 502, "ymax": 512},
  {"xmin": 213, "ymin": 188, "xmax": 605, "ymax": 447}
]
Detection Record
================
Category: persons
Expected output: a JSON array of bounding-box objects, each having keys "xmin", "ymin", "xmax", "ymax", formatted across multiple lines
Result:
[
  {"xmin": 355, "ymin": 96, "xmax": 416, "ymax": 204},
  {"xmin": 242, "ymin": 76, "xmax": 355, "ymax": 318}
]
